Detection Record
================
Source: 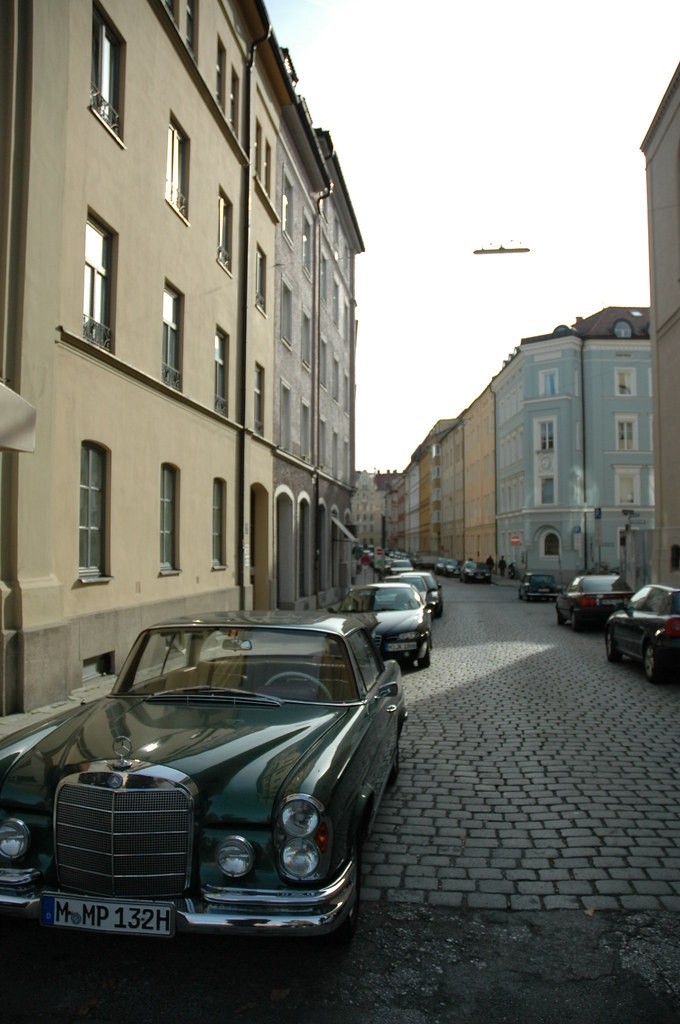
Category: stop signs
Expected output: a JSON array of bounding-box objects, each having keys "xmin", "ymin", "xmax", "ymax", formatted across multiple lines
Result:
[
  {"xmin": 511, "ymin": 535, "xmax": 520, "ymax": 545},
  {"xmin": 373, "ymin": 545, "xmax": 385, "ymax": 559},
  {"xmin": 360, "ymin": 555, "xmax": 371, "ymax": 566}
]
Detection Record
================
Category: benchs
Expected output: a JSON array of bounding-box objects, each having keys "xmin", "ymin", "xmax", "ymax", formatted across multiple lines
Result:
[{"xmin": 196, "ymin": 658, "xmax": 352, "ymax": 700}]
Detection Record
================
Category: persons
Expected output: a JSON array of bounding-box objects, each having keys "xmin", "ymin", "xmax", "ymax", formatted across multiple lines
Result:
[
  {"xmin": 485, "ymin": 555, "xmax": 494, "ymax": 572},
  {"xmin": 498, "ymin": 556, "xmax": 506, "ymax": 576}
]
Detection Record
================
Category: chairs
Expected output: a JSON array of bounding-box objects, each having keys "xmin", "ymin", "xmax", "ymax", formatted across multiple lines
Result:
[
  {"xmin": 394, "ymin": 591, "xmax": 413, "ymax": 608},
  {"xmin": 286, "ymin": 678, "xmax": 351, "ymax": 699},
  {"xmin": 202, "ymin": 673, "xmax": 248, "ymax": 691}
]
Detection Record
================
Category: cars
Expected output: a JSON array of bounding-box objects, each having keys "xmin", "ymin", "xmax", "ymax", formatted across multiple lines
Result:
[
  {"xmin": 360, "ymin": 548, "xmax": 463, "ymax": 621},
  {"xmin": 327, "ymin": 582, "xmax": 437, "ymax": 669},
  {"xmin": 517, "ymin": 572, "xmax": 563, "ymax": 603},
  {"xmin": 458, "ymin": 561, "xmax": 493, "ymax": 584},
  {"xmin": 602, "ymin": 581, "xmax": 680, "ymax": 687},
  {"xmin": 553, "ymin": 573, "xmax": 636, "ymax": 633},
  {"xmin": 0, "ymin": 608, "xmax": 408, "ymax": 952}
]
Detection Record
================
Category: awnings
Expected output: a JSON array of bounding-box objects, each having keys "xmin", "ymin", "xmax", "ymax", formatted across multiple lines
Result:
[
  {"xmin": 0, "ymin": 382, "xmax": 39, "ymax": 453},
  {"xmin": 331, "ymin": 517, "xmax": 360, "ymax": 544}
]
